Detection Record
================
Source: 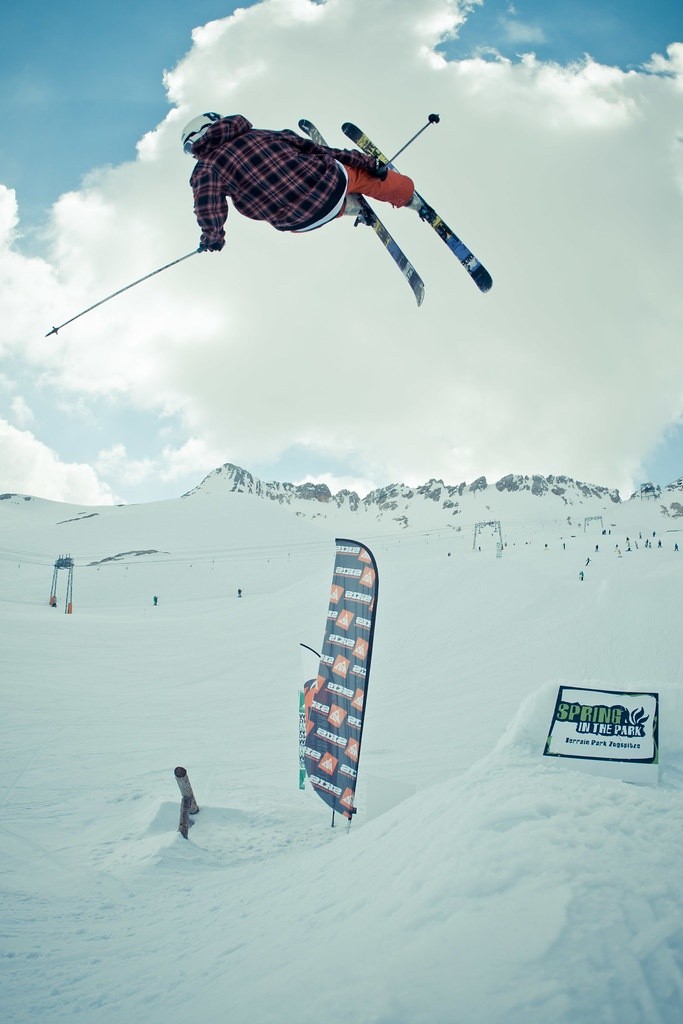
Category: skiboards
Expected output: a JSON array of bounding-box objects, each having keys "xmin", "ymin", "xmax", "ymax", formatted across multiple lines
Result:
[{"xmin": 297, "ymin": 117, "xmax": 495, "ymax": 307}]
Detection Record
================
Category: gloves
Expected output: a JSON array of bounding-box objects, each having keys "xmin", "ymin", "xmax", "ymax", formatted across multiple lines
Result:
[
  {"xmin": 369, "ymin": 157, "xmax": 387, "ymax": 182},
  {"xmin": 200, "ymin": 239, "xmax": 225, "ymax": 252}
]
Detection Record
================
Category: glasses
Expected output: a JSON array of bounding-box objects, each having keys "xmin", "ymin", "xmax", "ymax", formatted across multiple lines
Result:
[{"xmin": 183, "ymin": 140, "xmax": 196, "ymax": 158}]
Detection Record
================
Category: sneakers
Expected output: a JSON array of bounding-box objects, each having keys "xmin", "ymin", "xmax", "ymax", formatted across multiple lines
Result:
[
  {"xmin": 404, "ymin": 194, "xmax": 422, "ymax": 211},
  {"xmin": 343, "ymin": 192, "xmax": 362, "ymax": 216}
]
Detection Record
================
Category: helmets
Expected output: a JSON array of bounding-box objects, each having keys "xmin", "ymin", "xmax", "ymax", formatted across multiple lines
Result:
[{"xmin": 180, "ymin": 112, "xmax": 222, "ymax": 143}]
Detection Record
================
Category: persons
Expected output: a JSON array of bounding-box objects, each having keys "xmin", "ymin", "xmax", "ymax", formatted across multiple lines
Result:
[
  {"xmin": 446, "ymin": 529, "xmax": 679, "ymax": 582},
  {"xmin": 154, "ymin": 596, "xmax": 158, "ymax": 605},
  {"xmin": 181, "ymin": 111, "xmax": 425, "ymax": 252},
  {"xmin": 238, "ymin": 589, "xmax": 242, "ymax": 597},
  {"xmin": 52, "ymin": 596, "xmax": 57, "ymax": 607}
]
